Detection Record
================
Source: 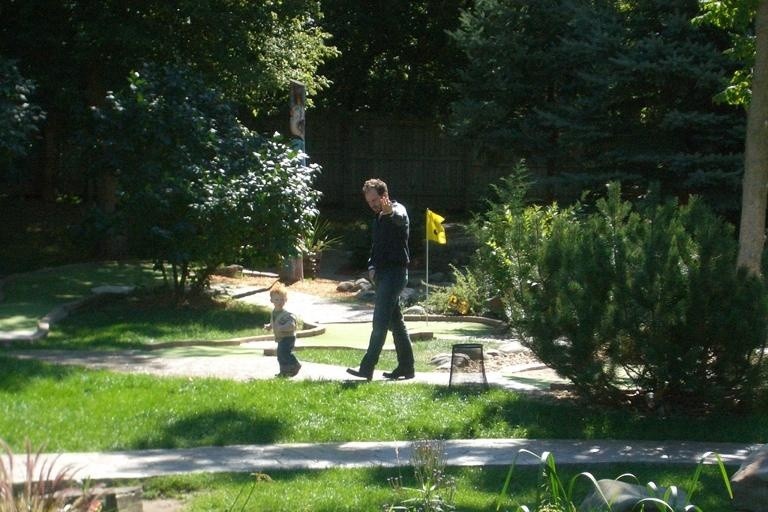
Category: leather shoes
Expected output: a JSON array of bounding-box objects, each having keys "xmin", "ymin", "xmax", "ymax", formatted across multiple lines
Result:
[
  {"xmin": 346, "ymin": 368, "xmax": 373, "ymax": 381},
  {"xmin": 383, "ymin": 368, "xmax": 415, "ymax": 379}
]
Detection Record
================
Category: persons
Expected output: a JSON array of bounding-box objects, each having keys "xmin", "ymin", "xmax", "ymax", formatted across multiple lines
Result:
[
  {"xmin": 345, "ymin": 177, "xmax": 414, "ymax": 381},
  {"xmin": 262, "ymin": 279, "xmax": 302, "ymax": 378}
]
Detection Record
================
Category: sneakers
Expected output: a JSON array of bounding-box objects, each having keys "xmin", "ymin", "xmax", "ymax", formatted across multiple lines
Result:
[{"xmin": 275, "ymin": 362, "xmax": 301, "ymax": 377}]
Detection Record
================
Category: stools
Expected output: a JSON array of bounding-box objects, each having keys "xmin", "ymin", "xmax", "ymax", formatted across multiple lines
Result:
[{"xmin": 446, "ymin": 342, "xmax": 491, "ymax": 393}]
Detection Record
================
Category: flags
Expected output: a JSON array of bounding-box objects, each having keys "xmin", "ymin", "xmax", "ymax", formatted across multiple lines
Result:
[{"xmin": 424, "ymin": 210, "xmax": 447, "ymax": 246}]
